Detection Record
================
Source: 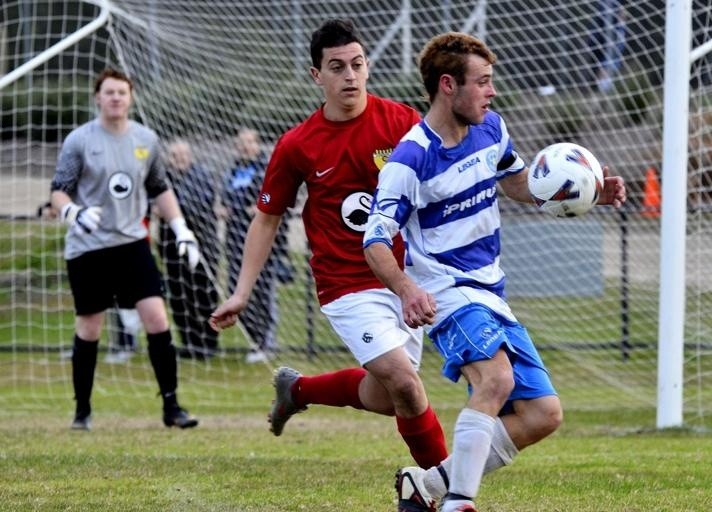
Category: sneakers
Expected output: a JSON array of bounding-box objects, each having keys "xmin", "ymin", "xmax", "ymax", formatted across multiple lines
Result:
[
  {"xmin": 72, "ymin": 398, "xmax": 92, "ymax": 427},
  {"xmin": 267, "ymin": 367, "xmax": 307, "ymax": 436},
  {"xmin": 162, "ymin": 398, "xmax": 198, "ymax": 428},
  {"xmin": 395, "ymin": 467, "xmax": 436, "ymax": 511}
]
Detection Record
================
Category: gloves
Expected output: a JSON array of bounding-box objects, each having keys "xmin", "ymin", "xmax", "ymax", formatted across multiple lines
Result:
[
  {"xmin": 175, "ymin": 227, "xmax": 200, "ymax": 273},
  {"xmin": 60, "ymin": 203, "xmax": 101, "ymax": 233}
]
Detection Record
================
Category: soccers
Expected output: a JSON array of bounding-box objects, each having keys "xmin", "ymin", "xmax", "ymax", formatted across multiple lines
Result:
[{"xmin": 527, "ymin": 142, "xmax": 604, "ymax": 216}]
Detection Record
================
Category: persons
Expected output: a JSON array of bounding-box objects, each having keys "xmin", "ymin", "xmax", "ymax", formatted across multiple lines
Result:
[
  {"xmin": 102, "ymin": 106, "xmax": 153, "ymax": 366},
  {"xmin": 361, "ymin": 32, "xmax": 626, "ymax": 512},
  {"xmin": 49, "ymin": 71, "xmax": 202, "ymax": 429},
  {"xmin": 206, "ymin": 18, "xmax": 448, "ymax": 471},
  {"xmin": 213, "ymin": 125, "xmax": 298, "ymax": 364},
  {"xmin": 157, "ymin": 138, "xmax": 220, "ymax": 362}
]
